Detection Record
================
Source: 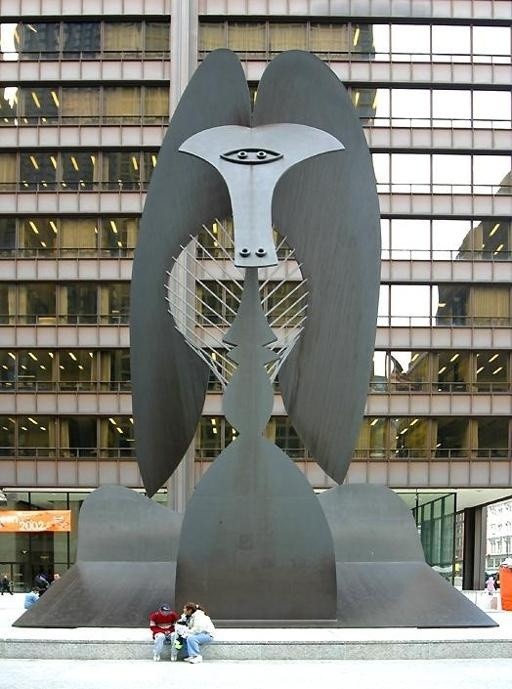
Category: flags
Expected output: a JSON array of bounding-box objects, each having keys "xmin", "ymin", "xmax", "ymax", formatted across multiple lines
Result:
[{"xmin": 388, "ymin": 353, "xmax": 417, "ymax": 390}]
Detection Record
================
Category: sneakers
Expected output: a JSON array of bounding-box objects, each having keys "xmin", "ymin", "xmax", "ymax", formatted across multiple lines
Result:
[
  {"xmin": 170, "ymin": 651, "xmax": 177, "ymax": 661},
  {"xmin": 184, "ymin": 655, "xmax": 204, "ymax": 663},
  {"xmin": 153, "ymin": 652, "xmax": 160, "ymax": 661}
]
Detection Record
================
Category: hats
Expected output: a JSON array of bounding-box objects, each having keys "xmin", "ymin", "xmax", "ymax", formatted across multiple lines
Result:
[{"xmin": 159, "ymin": 603, "xmax": 171, "ymax": 612}]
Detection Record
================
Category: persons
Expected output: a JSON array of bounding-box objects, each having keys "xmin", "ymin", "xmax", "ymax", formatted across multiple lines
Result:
[
  {"xmin": 485, "ymin": 576, "xmax": 496, "ymax": 595},
  {"xmin": 50, "ymin": 573, "xmax": 61, "ymax": 584},
  {"xmin": 24, "ymin": 587, "xmax": 40, "ymax": 609},
  {"xmin": 176, "ymin": 601, "xmax": 217, "ymax": 664},
  {"xmin": 148, "ymin": 603, "xmax": 180, "ymax": 662},
  {"xmin": 1, "ymin": 575, "xmax": 14, "ymax": 596}
]
